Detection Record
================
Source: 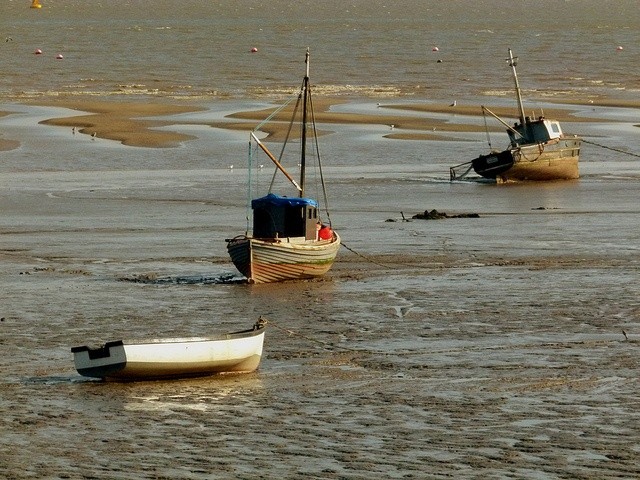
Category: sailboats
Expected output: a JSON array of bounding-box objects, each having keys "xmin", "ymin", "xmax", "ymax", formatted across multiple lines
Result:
[
  {"xmin": 473, "ymin": 49, "xmax": 581, "ymax": 178},
  {"xmin": 225, "ymin": 47, "xmax": 341, "ymax": 283}
]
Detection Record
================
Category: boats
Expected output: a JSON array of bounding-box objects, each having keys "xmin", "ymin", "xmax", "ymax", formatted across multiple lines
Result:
[{"xmin": 72, "ymin": 323, "xmax": 265, "ymax": 377}]
